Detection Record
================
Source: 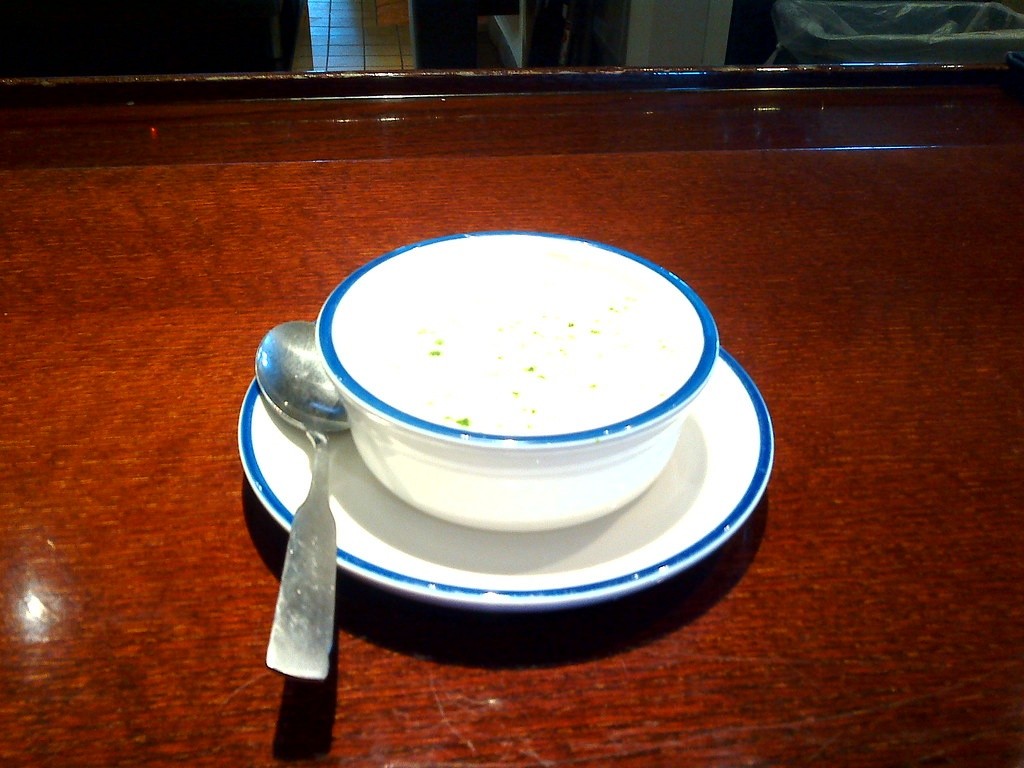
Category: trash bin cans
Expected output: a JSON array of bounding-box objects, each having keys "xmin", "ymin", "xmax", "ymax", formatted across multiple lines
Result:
[{"xmin": 770, "ymin": 0, "xmax": 1024, "ymax": 68}]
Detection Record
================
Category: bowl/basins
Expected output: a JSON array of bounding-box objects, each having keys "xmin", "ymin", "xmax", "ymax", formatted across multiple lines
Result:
[{"xmin": 316, "ymin": 230, "xmax": 719, "ymax": 530}]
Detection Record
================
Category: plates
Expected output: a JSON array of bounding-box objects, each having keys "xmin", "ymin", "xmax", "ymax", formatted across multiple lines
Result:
[{"xmin": 237, "ymin": 345, "xmax": 770, "ymax": 611}]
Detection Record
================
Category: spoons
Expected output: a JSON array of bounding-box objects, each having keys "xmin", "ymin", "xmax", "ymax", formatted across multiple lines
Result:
[{"xmin": 255, "ymin": 320, "xmax": 352, "ymax": 680}]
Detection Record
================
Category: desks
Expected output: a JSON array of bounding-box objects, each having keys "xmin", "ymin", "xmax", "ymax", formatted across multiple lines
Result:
[{"xmin": 0, "ymin": 59, "xmax": 1024, "ymax": 768}]
